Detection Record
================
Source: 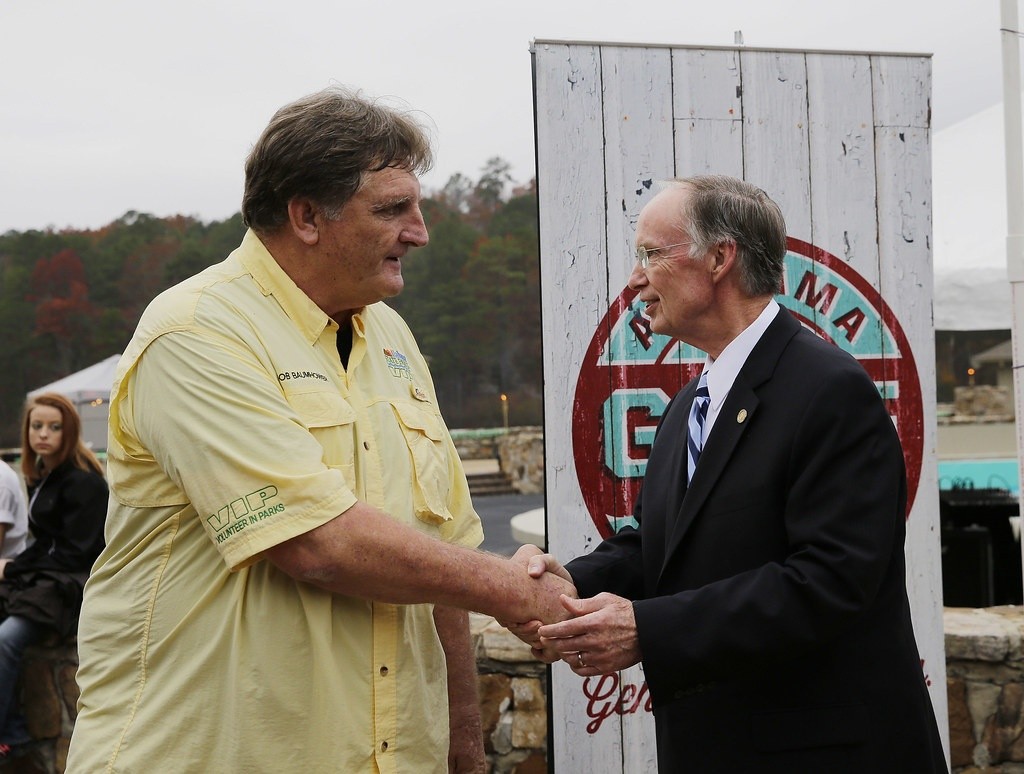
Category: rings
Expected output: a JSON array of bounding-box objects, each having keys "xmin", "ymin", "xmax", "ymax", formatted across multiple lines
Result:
[{"xmin": 578, "ymin": 653, "xmax": 586, "ymax": 668}]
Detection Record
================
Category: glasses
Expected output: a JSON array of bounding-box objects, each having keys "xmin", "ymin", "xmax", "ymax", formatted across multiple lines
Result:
[{"xmin": 634, "ymin": 241, "xmax": 696, "ymax": 268}]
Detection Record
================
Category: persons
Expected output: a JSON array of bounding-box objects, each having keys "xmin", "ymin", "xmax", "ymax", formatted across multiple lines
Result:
[
  {"xmin": 0, "ymin": 394, "xmax": 110, "ymax": 774},
  {"xmin": 500, "ymin": 177, "xmax": 950, "ymax": 774},
  {"xmin": 62, "ymin": 90, "xmax": 576, "ymax": 774}
]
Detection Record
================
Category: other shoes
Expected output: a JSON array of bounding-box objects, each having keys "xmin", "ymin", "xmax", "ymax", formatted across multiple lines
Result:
[{"xmin": 0, "ymin": 741, "xmax": 38, "ymax": 764}]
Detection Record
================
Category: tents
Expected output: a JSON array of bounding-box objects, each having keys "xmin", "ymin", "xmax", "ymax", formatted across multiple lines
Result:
[{"xmin": 28, "ymin": 353, "xmax": 123, "ymax": 453}]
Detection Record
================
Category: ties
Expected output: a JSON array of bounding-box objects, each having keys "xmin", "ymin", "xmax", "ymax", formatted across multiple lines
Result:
[{"xmin": 688, "ymin": 371, "xmax": 712, "ymax": 484}]
